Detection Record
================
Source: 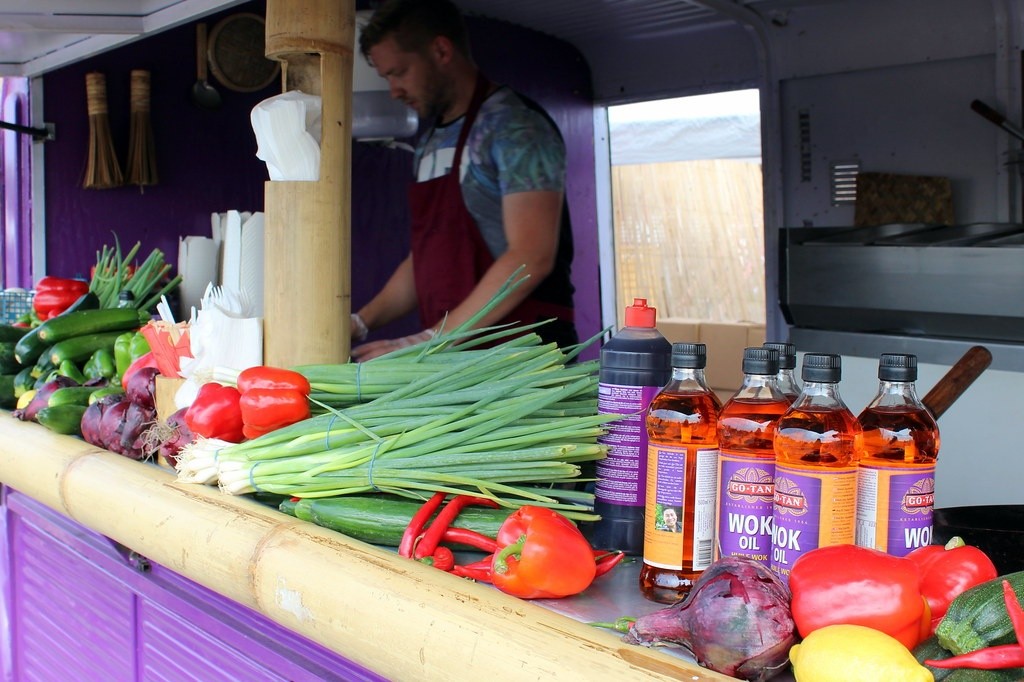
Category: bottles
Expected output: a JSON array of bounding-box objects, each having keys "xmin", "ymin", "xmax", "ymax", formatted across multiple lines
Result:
[
  {"xmin": 768, "ymin": 352, "xmax": 864, "ymax": 584},
  {"xmin": 591, "ymin": 298, "xmax": 672, "ymax": 556},
  {"xmin": 712, "ymin": 347, "xmax": 792, "ymax": 571},
  {"xmin": 856, "ymin": 353, "xmax": 942, "ymax": 558},
  {"xmin": 761, "ymin": 342, "xmax": 802, "ymax": 406},
  {"xmin": 639, "ymin": 342, "xmax": 723, "ymax": 604}
]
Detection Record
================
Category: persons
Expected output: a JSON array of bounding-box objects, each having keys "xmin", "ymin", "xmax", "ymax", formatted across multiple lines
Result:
[{"xmin": 350, "ymin": 1, "xmax": 580, "ymax": 369}]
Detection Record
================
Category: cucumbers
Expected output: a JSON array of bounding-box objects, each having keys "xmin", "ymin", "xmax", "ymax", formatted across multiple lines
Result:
[{"xmin": 1, "ymin": 292, "xmax": 141, "ymax": 408}]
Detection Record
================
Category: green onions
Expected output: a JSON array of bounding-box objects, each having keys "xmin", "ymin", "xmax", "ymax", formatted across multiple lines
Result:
[
  {"xmin": 88, "ymin": 229, "xmax": 183, "ymax": 311},
  {"xmin": 172, "ymin": 265, "xmax": 644, "ymax": 523}
]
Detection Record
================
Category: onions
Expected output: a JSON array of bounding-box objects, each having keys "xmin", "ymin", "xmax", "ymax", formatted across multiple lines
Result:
[
  {"xmin": 13, "ymin": 366, "xmax": 195, "ymax": 470},
  {"xmin": 627, "ymin": 558, "xmax": 797, "ymax": 681}
]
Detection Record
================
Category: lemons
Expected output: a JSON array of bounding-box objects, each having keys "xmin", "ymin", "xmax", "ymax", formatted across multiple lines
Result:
[{"xmin": 788, "ymin": 626, "xmax": 937, "ymax": 682}]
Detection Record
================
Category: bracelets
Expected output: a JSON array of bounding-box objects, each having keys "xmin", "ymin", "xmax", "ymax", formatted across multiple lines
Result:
[{"xmin": 351, "ymin": 312, "xmax": 368, "ymax": 342}]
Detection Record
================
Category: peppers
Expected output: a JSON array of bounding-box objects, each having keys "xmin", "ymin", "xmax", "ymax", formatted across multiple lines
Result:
[
  {"xmin": 397, "ymin": 491, "xmax": 626, "ymax": 599},
  {"xmin": 786, "ymin": 534, "xmax": 1024, "ymax": 668},
  {"xmin": 14, "ymin": 274, "xmax": 88, "ymax": 327}
]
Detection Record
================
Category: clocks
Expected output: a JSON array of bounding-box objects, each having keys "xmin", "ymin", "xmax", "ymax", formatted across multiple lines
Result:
[{"xmin": 207, "ymin": 13, "xmax": 281, "ymax": 93}]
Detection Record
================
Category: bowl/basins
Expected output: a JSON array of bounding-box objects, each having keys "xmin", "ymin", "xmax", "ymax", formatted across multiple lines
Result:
[{"xmin": 932, "ymin": 504, "xmax": 1024, "ymax": 576}]
